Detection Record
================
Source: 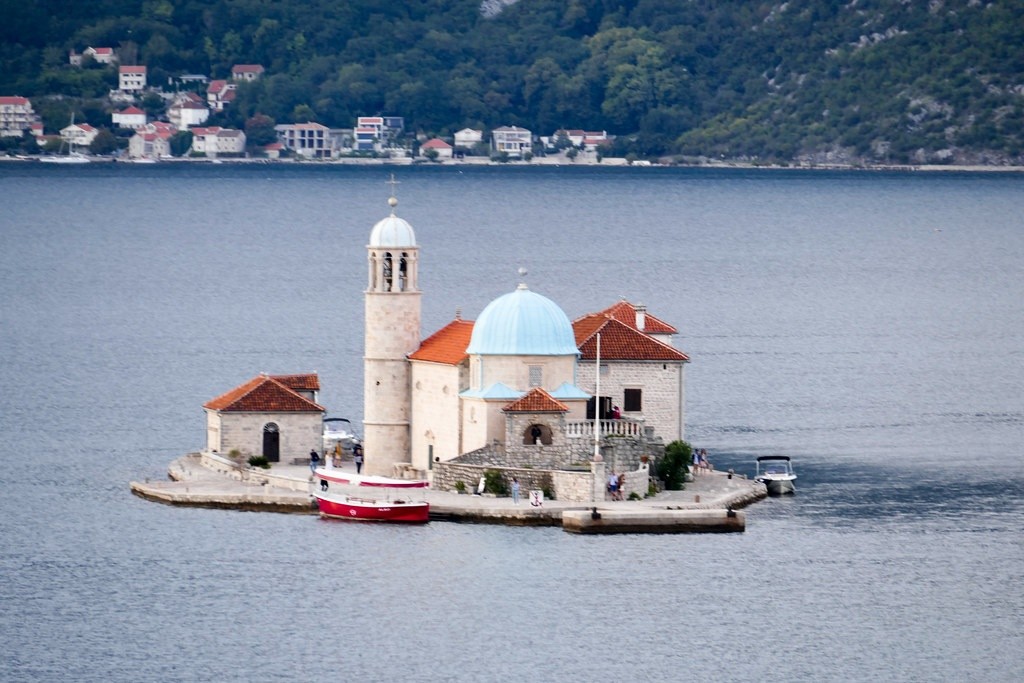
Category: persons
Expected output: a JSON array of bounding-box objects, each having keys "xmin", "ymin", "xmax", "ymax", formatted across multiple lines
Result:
[
  {"xmin": 692, "ymin": 448, "xmax": 710, "ymax": 479},
  {"xmin": 510, "ymin": 477, "xmax": 520, "ymax": 505},
  {"xmin": 607, "ymin": 471, "xmax": 627, "ymax": 502},
  {"xmin": 310, "ymin": 440, "xmax": 364, "ymax": 474}
]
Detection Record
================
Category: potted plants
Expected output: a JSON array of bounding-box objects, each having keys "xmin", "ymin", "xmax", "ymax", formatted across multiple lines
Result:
[
  {"xmin": 480, "ymin": 469, "xmax": 507, "ymax": 497},
  {"xmin": 449, "ymin": 480, "xmax": 467, "ymax": 494}
]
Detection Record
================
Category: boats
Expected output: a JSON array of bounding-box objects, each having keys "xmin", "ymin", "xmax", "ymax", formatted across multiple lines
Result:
[
  {"xmin": 322, "ymin": 417, "xmax": 359, "ymax": 454},
  {"xmin": 754, "ymin": 455, "xmax": 798, "ymax": 495},
  {"xmin": 311, "ymin": 468, "xmax": 430, "ymax": 525}
]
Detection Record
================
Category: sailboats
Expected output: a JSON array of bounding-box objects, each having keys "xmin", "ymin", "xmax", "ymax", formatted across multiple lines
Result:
[{"xmin": 38, "ymin": 112, "xmax": 92, "ymax": 164}]
